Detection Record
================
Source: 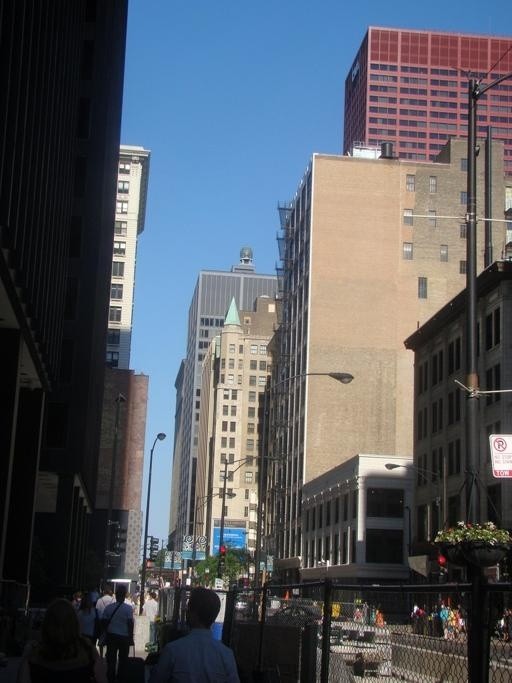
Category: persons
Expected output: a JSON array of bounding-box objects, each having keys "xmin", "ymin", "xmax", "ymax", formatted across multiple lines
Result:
[
  {"xmin": 351, "ymin": 599, "xmax": 386, "ymax": 628},
  {"xmin": 0, "ymin": 583, "xmax": 160, "ymax": 683},
  {"xmin": 151, "ymin": 585, "xmax": 241, "ymax": 683},
  {"xmin": 411, "ymin": 602, "xmax": 512, "ymax": 643}
]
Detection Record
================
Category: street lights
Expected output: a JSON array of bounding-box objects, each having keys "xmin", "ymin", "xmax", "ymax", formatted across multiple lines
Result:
[
  {"xmin": 190, "ymin": 492, "xmax": 237, "ymax": 587},
  {"xmin": 386, "ymin": 461, "xmax": 445, "ymax": 528},
  {"xmin": 253, "ymin": 372, "xmax": 355, "ymax": 612},
  {"xmin": 240, "ymin": 529, "xmax": 271, "ymax": 583},
  {"xmin": 217, "ymin": 454, "xmax": 282, "ymax": 583},
  {"xmin": 138, "ymin": 432, "xmax": 167, "ymax": 616},
  {"xmin": 101, "ymin": 393, "xmax": 129, "ymax": 593}
]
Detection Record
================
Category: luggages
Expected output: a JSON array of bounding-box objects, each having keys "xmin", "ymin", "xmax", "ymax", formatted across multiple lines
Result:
[{"xmin": 126, "ymin": 657, "xmax": 146, "ymax": 683}]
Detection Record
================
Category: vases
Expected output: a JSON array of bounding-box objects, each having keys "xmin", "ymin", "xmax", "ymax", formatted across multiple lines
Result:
[{"xmin": 439, "ymin": 540, "xmax": 512, "ymax": 566}]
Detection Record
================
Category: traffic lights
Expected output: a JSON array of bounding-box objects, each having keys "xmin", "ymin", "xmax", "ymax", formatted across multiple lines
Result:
[
  {"xmin": 114, "ymin": 527, "xmax": 126, "ymax": 552},
  {"xmin": 151, "ymin": 537, "xmax": 159, "ymax": 559},
  {"xmin": 219, "ymin": 546, "xmax": 225, "ymax": 565},
  {"xmin": 437, "ymin": 549, "xmax": 448, "ymax": 575}
]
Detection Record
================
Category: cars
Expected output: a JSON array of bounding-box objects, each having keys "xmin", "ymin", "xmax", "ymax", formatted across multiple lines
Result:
[
  {"xmin": 233, "ymin": 598, "xmax": 253, "ymax": 622},
  {"xmin": 146, "ymin": 584, "xmax": 159, "ymax": 598},
  {"xmin": 265, "ymin": 595, "xmax": 282, "ymax": 615},
  {"xmin": 275, "ymin": 604, "xmax": 392, "ymax": 670}
]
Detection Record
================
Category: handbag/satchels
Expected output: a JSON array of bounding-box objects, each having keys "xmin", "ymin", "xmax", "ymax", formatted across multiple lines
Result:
[{"xmin": 93, "ymin": 617, "xmax": 111, "ymax": 646}]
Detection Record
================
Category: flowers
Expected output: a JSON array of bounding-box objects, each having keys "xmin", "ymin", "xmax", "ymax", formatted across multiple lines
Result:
[{"xmin": 432, "ymin": 521, "xmax": 512, "ymax": 544}]
